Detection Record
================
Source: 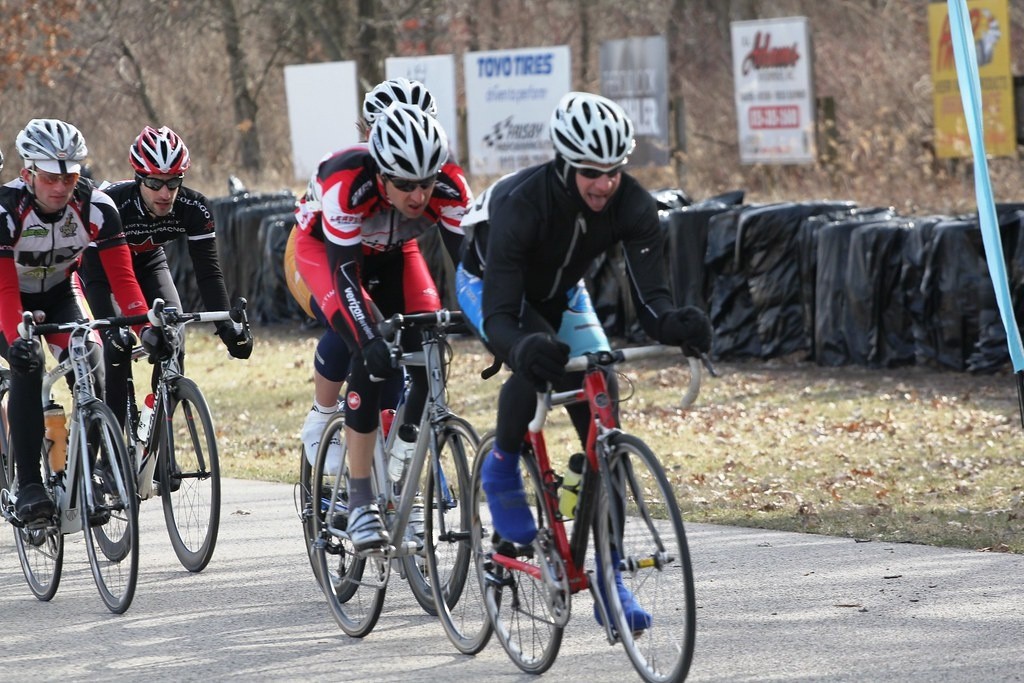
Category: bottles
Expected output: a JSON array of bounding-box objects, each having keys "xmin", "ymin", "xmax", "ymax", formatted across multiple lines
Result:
[
  {"xmin": 559, "ymin": 453, "xmax": 585, "ymax": 517},
  {"xmin": 381, "ymin": 408, "xmax": 396, "ymax": 443},
  {"xmin": 42, "ymin": 400, "xmax": 68, "ymax": 472},
  {"xmin": 137, "ymin": 394, "xmax": 154, "ymax": 442},
  {"xmin": 387, "ymin": 424, "xmax": 419, "ymax": 481}
]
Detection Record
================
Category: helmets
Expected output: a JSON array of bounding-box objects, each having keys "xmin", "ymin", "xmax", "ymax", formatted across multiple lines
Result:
[
  {"xmin": 368, "ymin": 102, "xmax": 448, "ymax": 181},
  {"xmin": 16, "ymin": 118, "xmax": 89, "ymax": 160},
  {"xmin": 362, "ymin": 77, "xmax": 437, "ymax": 124},
  {"xmin": 549, "ymin": 92, "xmax": 634, "ymax": 164},
  {"xmin": 127, "ymin": 125, "xmax": 191, "ymax": 175}
]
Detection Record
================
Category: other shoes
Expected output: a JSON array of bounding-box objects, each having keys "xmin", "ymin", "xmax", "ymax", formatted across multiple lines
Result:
[
  {"xmin": 153, "ymin": 453, "xmax": 181, "ymax": 491},
  {"xmin": 15, "ymin": 481, "xmax": 55, "ymax": 522},
  {"xmin": 591, "ymin": 552, "xmax": 652, "ymax": 631},
  {"xmin": 476, "ymin": 441, "xmax": 538, "ymax": 544},
  {"xmin": 83, "ymin": 484, "xmax": 109, "ymax": 526}
]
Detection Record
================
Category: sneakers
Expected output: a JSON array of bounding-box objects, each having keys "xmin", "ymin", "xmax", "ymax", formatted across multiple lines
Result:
[
  {"xmin": 299, "ymin": 405, "xmax": 347, "ymax": 477},
  {"xmin": 390, "ymin": 480, "xmax": 425, "ymax": 540},
  {"xmin": 345, "ymin": 505, "xmax": 391, "ymax": 552},
  {"xmin": 387, "ymin": 432, "xmax": 420, "ymax": 482}
]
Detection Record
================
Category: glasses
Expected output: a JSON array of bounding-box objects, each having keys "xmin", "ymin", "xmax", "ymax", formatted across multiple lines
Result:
[
  {"xmin": 560, "ymin": 154, "xmax": 630, "ymax": 178},
  {"xmin": 135, "ymin": 171, "xmax": 185, "ymax": 192},
  {"xmin": 381, "ymin": 172, "xmax": 438, "ymax": 193},
  {"xmin": 27, "ymin": 168, "xmax": 78, "ymax": 185}
]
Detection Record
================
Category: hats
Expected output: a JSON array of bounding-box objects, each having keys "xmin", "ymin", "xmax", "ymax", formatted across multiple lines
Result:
[{"xmin": 25, "ymin": 157, "xmax": 82, "ymax": 175}]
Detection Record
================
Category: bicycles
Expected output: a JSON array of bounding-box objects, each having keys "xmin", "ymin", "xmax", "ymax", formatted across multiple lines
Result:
[
  {"xmin": 465, "ymin": 338, "xmax": 698, "ymax": 682},
  {"xmin": 293, "ymin": 428, "xmax": 474, "ymax": 618},
  {"xmin": 89, "ymin": 294, "xmax": 250, "ymax": 576},
  {"xmin": 305, "ymin": 311, "xmax": 503, "ymax": 659},
  {"xmin": 1, "ymin": 296, "xmax": 167, "ymax": 614}
]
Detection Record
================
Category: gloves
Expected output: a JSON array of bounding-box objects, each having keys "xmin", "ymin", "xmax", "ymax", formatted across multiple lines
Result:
[
  {"xmin": 659, "ymin": 306, "xmax": 716, "ymax": 358},
  {"xmin": 352, "ymin": 337, "xmax": 401, "ymax": 381},
  {"xmin": 222, "ymin": 322, "xmax": 254, "ymax": 359},
  {"xmin": 8, "ymin": 337, "xmax": 37, "ymax": 377},
  {"xmin": 511, "ymin": 329, "xmax": 570, "ymax": 391},
  {"xmin": 139, "ymin": 325, "xmax": 177, "ymax": 364}
]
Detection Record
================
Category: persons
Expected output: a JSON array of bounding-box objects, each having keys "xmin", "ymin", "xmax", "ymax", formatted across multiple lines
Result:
[
  {"xmin": 78, "ymin": 125, "xmax": 253, "ymax": 496},
  {"xmin": 454, "ymin": 91, "xmax": 713, "ymax": 628},
  {"xmin": 0, "ymin": 118, "xmax": 172, "ymax": 526},
  {"xmin": 283, "ymin": 79, "xmax": 475, "ymax": 552}
]
